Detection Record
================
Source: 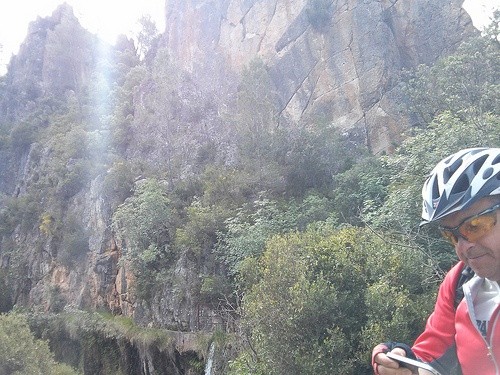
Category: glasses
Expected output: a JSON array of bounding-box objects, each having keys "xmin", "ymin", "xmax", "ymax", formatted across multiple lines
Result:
[{"xmin": 437, "ymin": 204, "xmax": 499, "ymax": 242}]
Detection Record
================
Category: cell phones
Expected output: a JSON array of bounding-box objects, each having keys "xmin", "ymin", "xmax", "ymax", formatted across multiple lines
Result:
[{"xmin": 387, "ymin": 352, "xmax": 440, "ymax": 375}]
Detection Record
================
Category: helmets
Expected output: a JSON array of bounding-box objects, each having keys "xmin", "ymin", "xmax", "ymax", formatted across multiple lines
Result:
[{"xmin": 419, "ymin": 147, "xmax": 500, "ymax": 229}]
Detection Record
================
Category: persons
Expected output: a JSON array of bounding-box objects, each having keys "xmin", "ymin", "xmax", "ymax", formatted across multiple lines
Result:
[{"xmin": 371, "ymin": 147, "xmax": 500, "ymax": 375}]
